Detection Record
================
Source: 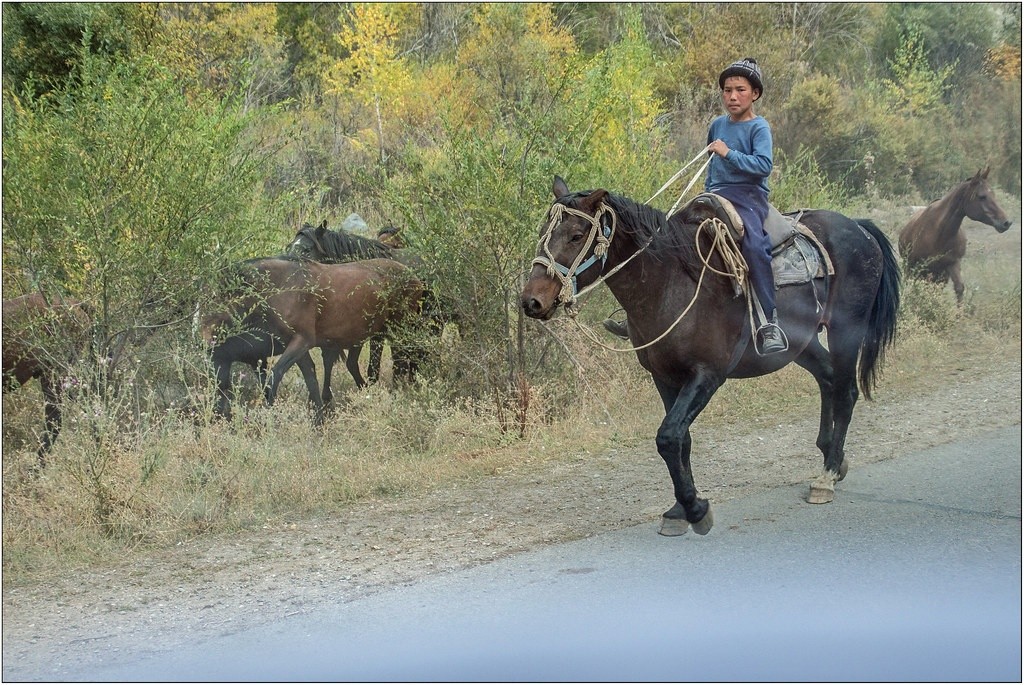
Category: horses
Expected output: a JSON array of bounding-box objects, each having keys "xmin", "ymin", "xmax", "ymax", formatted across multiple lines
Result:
[
  {"xmin": 206, "ymin": 220, "xmax": 463, "ymax": 431},
  {"xmin": 524, "ymin": 174, "xmax": 902, "ymax": 536},
  {"xmin": 1, "ymin": 291, "xmax": 96, "ymax": 473},
  {"xmin": 898, "ymin": 166, "xmax": 1012, "ymax": 309}
]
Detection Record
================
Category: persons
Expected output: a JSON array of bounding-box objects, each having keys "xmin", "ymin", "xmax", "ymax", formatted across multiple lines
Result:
[
  {"xmin": 601, "ymin": 57, "xmax": 787, "ymax": 354},
  {"xmin": 365, "ymin": 227, "xmax": 408, "ymax": 384}
]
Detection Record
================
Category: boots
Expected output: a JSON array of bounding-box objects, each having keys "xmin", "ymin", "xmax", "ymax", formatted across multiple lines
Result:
[
  {"xmin": 753, "ymin": 308, "xmax": 786, "ymax": 353},
  {"xmin": 602, "ymin": 318, "xmax": 628, "ymax": 337}
]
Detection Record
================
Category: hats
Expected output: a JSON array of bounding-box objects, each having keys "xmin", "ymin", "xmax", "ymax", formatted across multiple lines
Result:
[{"xmin": 718, "ymin": 58, "xmax": 763, "ymax": 102}]
